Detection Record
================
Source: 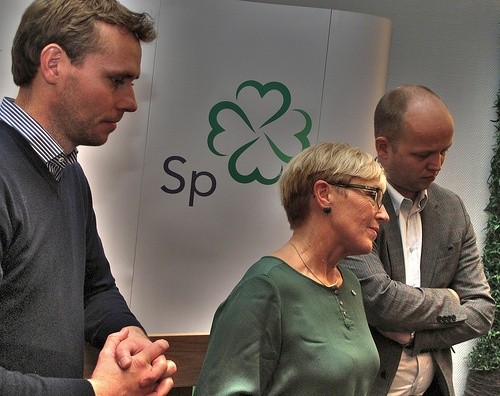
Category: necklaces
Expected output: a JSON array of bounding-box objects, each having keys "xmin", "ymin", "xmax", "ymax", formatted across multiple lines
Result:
[{"xmin": 288, "ymin": 238, "xmax": 339, "ymax": 288}]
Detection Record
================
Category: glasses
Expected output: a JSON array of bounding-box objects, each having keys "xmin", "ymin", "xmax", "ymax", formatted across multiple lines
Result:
[{"xmin": 326, "ymin": 181, "xmax": 383, "ymax": 209}]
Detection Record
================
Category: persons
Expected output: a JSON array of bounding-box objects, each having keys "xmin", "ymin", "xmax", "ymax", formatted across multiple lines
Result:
[
  {"xmin": 194, "ymin": 142, "xmax": 390, "ymax": 396},
  {"xmin": 333, "ymin": 84, "xmax": 494, "ymax": 396},
  {"xmin": 0, "ymin": 0, "xmax": 177, "ymax": 396}
]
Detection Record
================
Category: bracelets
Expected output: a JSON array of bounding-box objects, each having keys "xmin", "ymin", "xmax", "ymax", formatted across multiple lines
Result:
[{"xmin": 402, "ymin": 332, "xmax": 416, "ymax": 348}]
